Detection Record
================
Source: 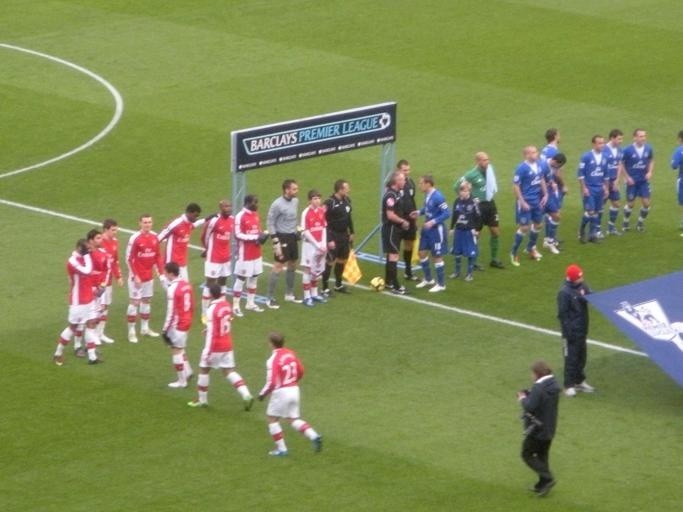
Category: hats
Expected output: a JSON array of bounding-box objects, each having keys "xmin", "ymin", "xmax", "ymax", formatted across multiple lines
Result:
[{"xmin": 566, "ymin": 264, "xmax": 583, "ymax": 282}]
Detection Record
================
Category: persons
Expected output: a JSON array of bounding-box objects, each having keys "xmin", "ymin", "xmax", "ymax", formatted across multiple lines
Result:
[
  {"xmin": 554, "ymin": 264, "xmax": 596, "ymax": 401},
  {"xmin": 201, "ymin": 179, "xmax": 358, "ymax": 316},
  {"xmin": 159, "ymin": 259, "xmax": 195, "ymax": 389},
  {"xmin": 51, "ymin": 202, "xmax": 217, "ymax": 365},
  {"xmin": 510, "ymin": 129, "xmax": 569, "ymax": 267},
  {"xmin": 258, "ymin": 331, "xmax": 325, "ymax": 454},
  {"xmin": 518, "ymin": 361, "xmax": 561, "ymax": 499},
  {"xmin": 578, "ymin": 130, "xmax": 683, "ymax": 242},
  {"xmin": 380, "ymin": 152, "xmax": 507, "ymax": 298},
  {"xmin": 187, "ymin": 278, "xmax": 254, "ymax": 411}
]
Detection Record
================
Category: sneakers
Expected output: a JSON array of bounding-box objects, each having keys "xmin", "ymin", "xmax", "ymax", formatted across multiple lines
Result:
[
  {"xmin": 564, "ymin": 381, "xmax": 594, "ymax": 396},
  {"xmin": 448, "ymin": 237, "xmax": 561, "ymax": 282},
  {"xmin": 381, "ymin": 273, "xmax": 448, "ymax": 294},
  {"xmin": 530, "ymin": 477, "xmax": 555, "ymax": 496},
  {"xmin": 168, "ymin": 367, "xmax": 192, "ymax": 388},
  {"xmin": 187, "ymin": 398, "xmax": 207, "ymax": 407},
  {"xmin": 54, "ymin": 333, "xmax": 114, "ymax": 365},
  {"xmin": 312, "ymin": 435, "xmax": 323, "ymax": 451},
  {"xmin": 141, "ymin": 329, "xmax": 159, "ymax": 336},
  {"xmin": 244, "ymin": 395, "xmax": 256, "ymax": 411},
  {"xmin": 231, "ymin": 286, "xmax": 351, "ymax": 317},
  {"xmin": 128, "ymin": 331, "xmax": 137, "ymax": 343},
  {"xmin": 268, "ymin": 448, "xmax": 287, "ymax": 456},
  {"xmin": 579, "ymin": 224, "xmax": 643, "ymax": 244}
]
